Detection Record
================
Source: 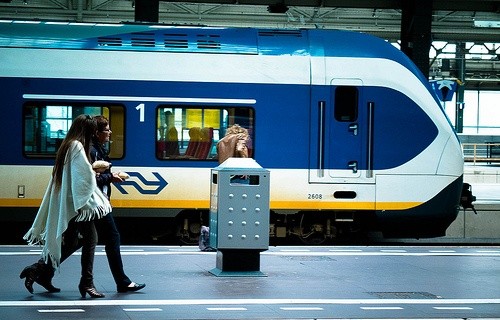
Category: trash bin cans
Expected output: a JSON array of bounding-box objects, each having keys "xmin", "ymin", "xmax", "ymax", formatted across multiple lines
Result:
[{"xmin": 209, "ymin": 157, "xmax": 271, "ymax": 277}]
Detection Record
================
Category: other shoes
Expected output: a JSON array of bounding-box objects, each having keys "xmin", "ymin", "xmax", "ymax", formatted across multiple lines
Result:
[
  {"xmin": 117, "ymin": 282, "xmax": 145, "ymax": 293},
  {"xmin": 35, "ymin": 277, "xmax": 60, "ymax": 293}
]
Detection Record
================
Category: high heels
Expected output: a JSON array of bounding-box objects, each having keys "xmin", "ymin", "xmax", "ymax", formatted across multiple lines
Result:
[
  {"xmin": 20, "ymin": 266, "xmax": 35, "ymax": 294},
  {"xmin": 78, "ymin": 283, "xmax": 105, "ymax": 298}
]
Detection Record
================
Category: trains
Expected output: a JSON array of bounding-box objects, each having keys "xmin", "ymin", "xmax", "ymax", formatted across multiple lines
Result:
[{"xmin": 0, "ymin": 10, "xmax": 477, "ymax": 247}]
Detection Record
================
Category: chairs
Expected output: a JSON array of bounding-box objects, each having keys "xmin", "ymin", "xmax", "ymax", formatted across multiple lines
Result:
[{"xmin": 185, "ymin": 126, "xmax": 213, "ymax": 159}]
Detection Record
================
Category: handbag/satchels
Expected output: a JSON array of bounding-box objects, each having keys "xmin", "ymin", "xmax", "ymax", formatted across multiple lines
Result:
[{"xmin": 198, "ymin": 226, "xmax": 211, "ymax": 250}]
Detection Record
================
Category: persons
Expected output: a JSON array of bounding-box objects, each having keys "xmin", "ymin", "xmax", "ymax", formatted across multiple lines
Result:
[
  {"xmin": 34, "ymin": 114, "xmax": 146, "ymax": 293},
  {"xmin": 20, "ymin": 114, "xmax": 112, "ymax": 298}
]
